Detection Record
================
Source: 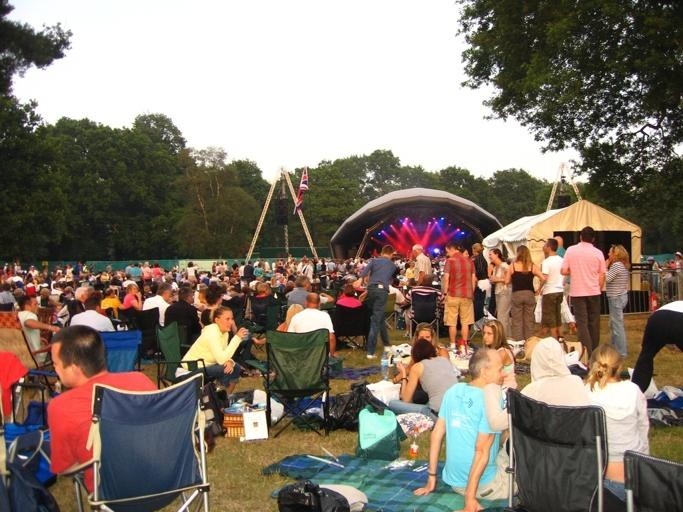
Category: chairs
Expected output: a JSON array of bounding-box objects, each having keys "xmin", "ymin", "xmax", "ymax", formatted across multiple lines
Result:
[
  {"xmin": 154, "ymin": 321, "xmax": 206, "ymax": 391},
  {"xmin": 383, "ymin": 293, "xmax": 397, "ymax": 333},
  {"xmin": 507, "ymin": 387, "xmax": 626, "ymax": 512},
  {"xmin": 410, "ymin": 289, "xmax": 440, "ymax": 341},
  {"xmin": 1, "ymin": 306, "xmax": 59, "ymax": 426},
  {"xmin": 623, "ymin": 449, "xmax": 683, "ymax": 512},
  {"xmin": 264, "ymin": 329, "xmax": 331, "ymax": 439},
  {"xmin": 178, "ymin": 324, "xmax": 190, "ymax": 354},
  {"xmin": 112, "ymin": 307, "xmax": 161, "ymax": 371},
  {"xmin": 319, "ymin": 289, "xmax": 337, "ymax": 311},
  {"xmin": 328, "ymin": 303, "xmax": 373, "ymax": 349},
  {"xmin": 100, "ymin": 331, "xmax": 143, "ymax": 374},
  {"xmin": 468, "ymin": 287, "xmax": 489, "ymax": 345}
]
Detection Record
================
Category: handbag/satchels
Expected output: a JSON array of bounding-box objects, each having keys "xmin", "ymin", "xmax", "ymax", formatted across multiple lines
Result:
[
  {"xmin": 0, "ymin": 420, "xmax": 62, "ymax": 512},
  {"xmin": 276, "ymin": 479, "xmax": 350, "ymax": 512},
  {"xmin": 627, "ymin": 365, "xmax": 683, "ymax": 428},
  {"xmin": 239, "ymin": 403, "xmax": 269, "ymax": 443},
  {"xmin": 355, "ymin": 404, "xmax": 407, "ymax": 461}
]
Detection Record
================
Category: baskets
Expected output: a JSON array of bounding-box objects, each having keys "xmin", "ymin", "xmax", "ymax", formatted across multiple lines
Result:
[{"xmin": 221, "ymin": 411, "xmax": 246, "ymax": 438}]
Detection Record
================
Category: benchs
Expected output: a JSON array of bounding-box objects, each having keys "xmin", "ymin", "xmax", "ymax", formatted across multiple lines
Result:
[{"xmin": 61, "ymin": 373, "xmax": 210, "ymax": 512}]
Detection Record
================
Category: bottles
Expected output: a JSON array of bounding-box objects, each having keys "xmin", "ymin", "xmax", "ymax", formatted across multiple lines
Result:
[
  {"xmin": 379, "ymin": 351, "xmax": 389, "ymax": 380},
  {"xmin": 408, "ymin": 427, "xmax": 420, "ymax": 460}
]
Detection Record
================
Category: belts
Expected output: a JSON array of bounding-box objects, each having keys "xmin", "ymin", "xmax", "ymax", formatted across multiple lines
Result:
[
  {"xmin": 426, "ymin": 406, "xmax": 440, "ymax": 418},
  {"xmin": 368, "ymin": 283, "xmax": 385, "ymax": 289}
]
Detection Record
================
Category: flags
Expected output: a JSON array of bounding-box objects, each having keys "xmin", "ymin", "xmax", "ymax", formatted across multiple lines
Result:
[{"xmin": 293, "ymin": 164, "xmax": 306, "ymax": 215}]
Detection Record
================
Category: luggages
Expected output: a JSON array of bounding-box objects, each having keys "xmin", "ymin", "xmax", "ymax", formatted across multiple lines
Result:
[{"xmin": 2, "ymin": 381, "xmax": 58, "ymax": 489}]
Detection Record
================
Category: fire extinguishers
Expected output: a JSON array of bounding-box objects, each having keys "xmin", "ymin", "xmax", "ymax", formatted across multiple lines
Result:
[{"xmin": 649, "ymin": 289, "xmax": 659, "ymax": 311}]
